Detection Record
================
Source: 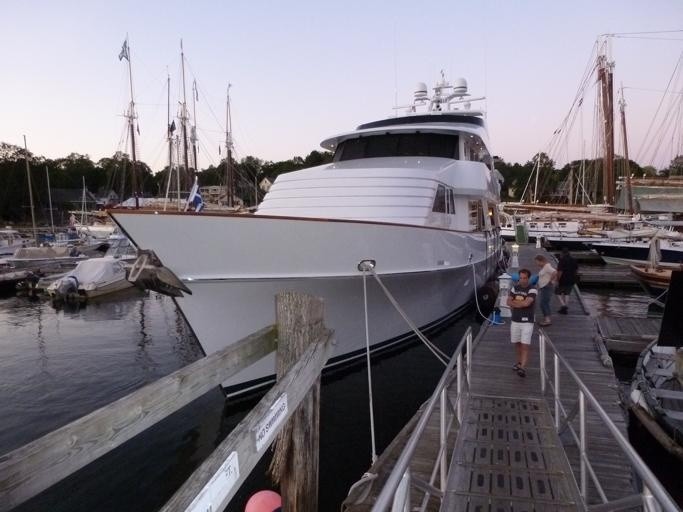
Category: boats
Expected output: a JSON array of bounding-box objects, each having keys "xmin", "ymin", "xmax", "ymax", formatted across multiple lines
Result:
[
  {"xmin": 32, "ymin": 253, "xmax": 137, "ymax": 304},
  {"xmin": 630, "ymin": 262, "xmax": 683, "ymax": 291},
  {"xmin": 627, "ymin": 331, "xmax": 683, "ymax": 456},
  {"xmin": 104, "ymin": 67, "xmax": 509, "ymax": 409},
  {"xmin": 0, "ymin": 133, "xmax": 62, "ymax": 283}
]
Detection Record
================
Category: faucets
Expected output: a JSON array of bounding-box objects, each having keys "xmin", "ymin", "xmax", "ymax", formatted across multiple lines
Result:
[{"xmin": 511, "ymin": 362, "xmax": 527, "ymax": 377}]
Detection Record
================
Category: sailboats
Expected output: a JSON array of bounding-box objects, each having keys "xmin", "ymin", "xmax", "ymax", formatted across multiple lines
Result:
[
  {"xmin": 497, "ymin": 35, "xmax": 680, "ymax": 272},
  {"xmin": 33, "ymin": 44, "xmax": 258, "ymax": 255}
]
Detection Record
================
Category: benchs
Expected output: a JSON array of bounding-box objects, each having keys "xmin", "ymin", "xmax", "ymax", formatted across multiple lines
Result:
[
  {"xmin": 189, "ymin": 184, "xmax": 204, "ymax": 213},
  {"xmin": 119, "ymin": 40, "xmax": 128, "ymax": 62}
]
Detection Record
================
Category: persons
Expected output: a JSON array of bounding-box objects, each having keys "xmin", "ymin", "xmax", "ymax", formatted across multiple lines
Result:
[{"xmin": 506, "ymin": 246, "xmax": 578, "ymax": 377}]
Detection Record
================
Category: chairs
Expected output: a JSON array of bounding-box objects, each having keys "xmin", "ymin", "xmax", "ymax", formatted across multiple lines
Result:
[{"xmin": 539, "ymin": 319, "xmax": 553, "ymax": 326}]
